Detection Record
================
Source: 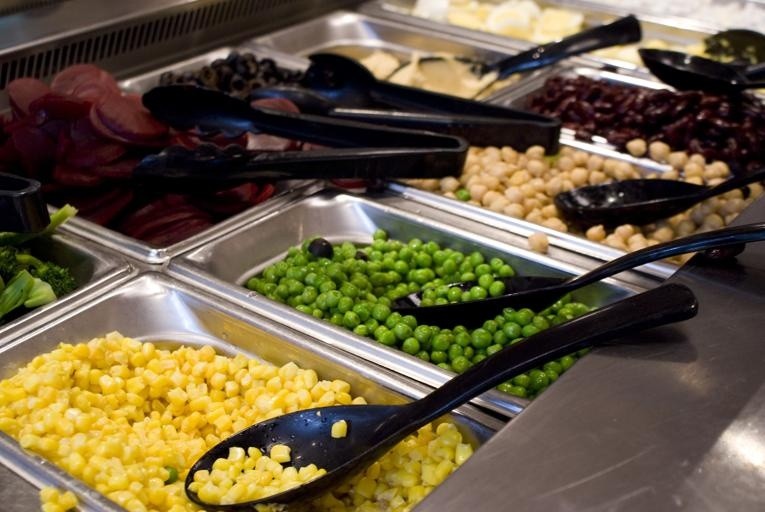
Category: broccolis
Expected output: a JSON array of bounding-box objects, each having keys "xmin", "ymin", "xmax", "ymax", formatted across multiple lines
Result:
[{"xmin": 0, "ymin": 201, "xmax": 80, "ymax": 319}]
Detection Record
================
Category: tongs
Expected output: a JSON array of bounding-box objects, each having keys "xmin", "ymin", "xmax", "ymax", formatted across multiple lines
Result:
[{"xmin": 137, "ymin": 52, "xmax": 557, "ymax": 186}]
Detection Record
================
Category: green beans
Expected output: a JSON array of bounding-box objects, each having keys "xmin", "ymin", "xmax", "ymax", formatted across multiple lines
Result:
[{"xmin": 244, "ymin": 140, "xmax": 762, "ymax": 399}]
